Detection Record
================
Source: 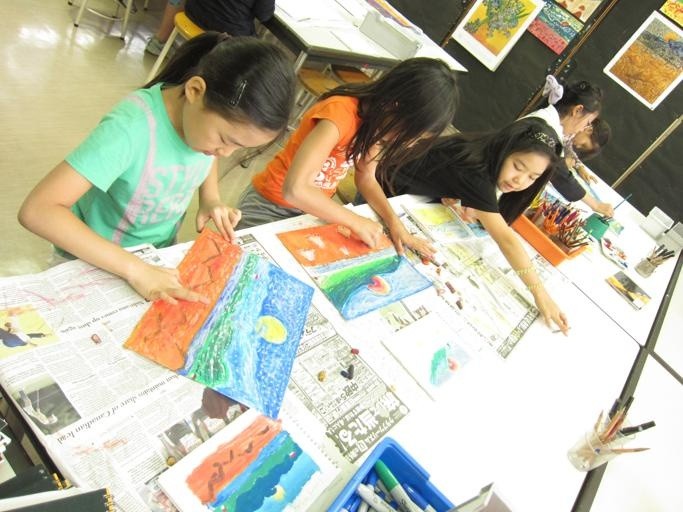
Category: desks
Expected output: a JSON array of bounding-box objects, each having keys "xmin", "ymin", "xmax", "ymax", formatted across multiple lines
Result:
[{"xmin": 260, "ymin": 0, "xmax": 468, "ymax": 74}]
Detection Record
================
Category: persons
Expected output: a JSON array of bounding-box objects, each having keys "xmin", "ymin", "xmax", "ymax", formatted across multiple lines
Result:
[
  {"xmin": 566, "ymin": 116, "xmax": 610, "ymax": 186},
  {"xmin": 235, "ymin": 56, "xmax": 462, "ymax": 263},
  {"xmin": 354, "ymin": 117, "xmax": 573, "ymax": 336},
  {"xmin": 18, "ymin": 387, "xmax": 58, "ymax": 426},
  {"xmin": 516, "ymin": 75, "xmax": 615, "ymax": 219},
  {"xmin": 145, "ymin": 0, "xmax": 185, "ymax": 58},
  {"xmin": 0, "ymin": 318, "xmax": 50, "ymax": 350},
  {"xmin": 20, "ymin": 34, "xmax": 297, "ymax": 307},
  {"xmin": 183, "ymin": 0, "xmax": 275, "ymax": 41}
]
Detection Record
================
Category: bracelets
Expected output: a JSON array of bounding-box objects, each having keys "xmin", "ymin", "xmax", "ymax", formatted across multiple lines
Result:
[
  {"xmin": 525, "ymin": 280, "xmax": 543, "ymax": 291},
  {"xmin": 517, "ymin": 265, "xmax": 538, "ymax": 277}
]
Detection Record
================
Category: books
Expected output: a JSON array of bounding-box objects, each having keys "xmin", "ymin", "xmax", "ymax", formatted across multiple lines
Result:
[{"xmin": 401, "ymin": 192, "xmax": 492, "ymax": 246}]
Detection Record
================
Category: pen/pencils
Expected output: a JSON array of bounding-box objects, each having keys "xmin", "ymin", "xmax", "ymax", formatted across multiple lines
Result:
[
  {"xmin": 593, "ymin": 396, "xmax": 658, "ymax": 455},
  {"xmin": 601, "ymin": 194, "xmax": 632, "ymax": 220},
  {"xmin": 650, "ymin": 243, "xmax": 675, "ymax": 266},
  {"xmin": 533, "ymin": 190, "xmax": 593, "ymax": 246},
  {"xmin": 341, "ymin": 460, "xmax": 440, "ymax": 512}
]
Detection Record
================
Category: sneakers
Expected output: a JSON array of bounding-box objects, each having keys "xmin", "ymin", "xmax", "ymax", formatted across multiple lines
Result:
[{"xmin": 144, "ymin": 33, "xmax": 180, "ymax": 59}]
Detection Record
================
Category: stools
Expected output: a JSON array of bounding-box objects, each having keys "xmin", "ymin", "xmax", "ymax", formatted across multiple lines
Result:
[
  {"xmin": 284, "ymin": 62, "xmax": 374, "ymax": 130},
  {"xmin": 65, "ymin": 0, "xmax": 136, "ymax": 41},
  {"xmin": 144, "ymin": 11, "xmax": 207, "ymax": 84}
]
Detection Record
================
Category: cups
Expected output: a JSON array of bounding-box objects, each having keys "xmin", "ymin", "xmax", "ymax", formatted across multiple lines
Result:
[
  {"xmin": 567, "ymin": 411, "xmax": 636, "ymax": 472},
  {"xmin": 634, "ymin": 259, "xmax": 656, "ymax": 277}
]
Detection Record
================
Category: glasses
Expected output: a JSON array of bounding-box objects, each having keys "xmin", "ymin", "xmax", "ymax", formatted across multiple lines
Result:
[{"xmin": 583, "ymin": 117, "xmax": 594, "ymax": 128}]
